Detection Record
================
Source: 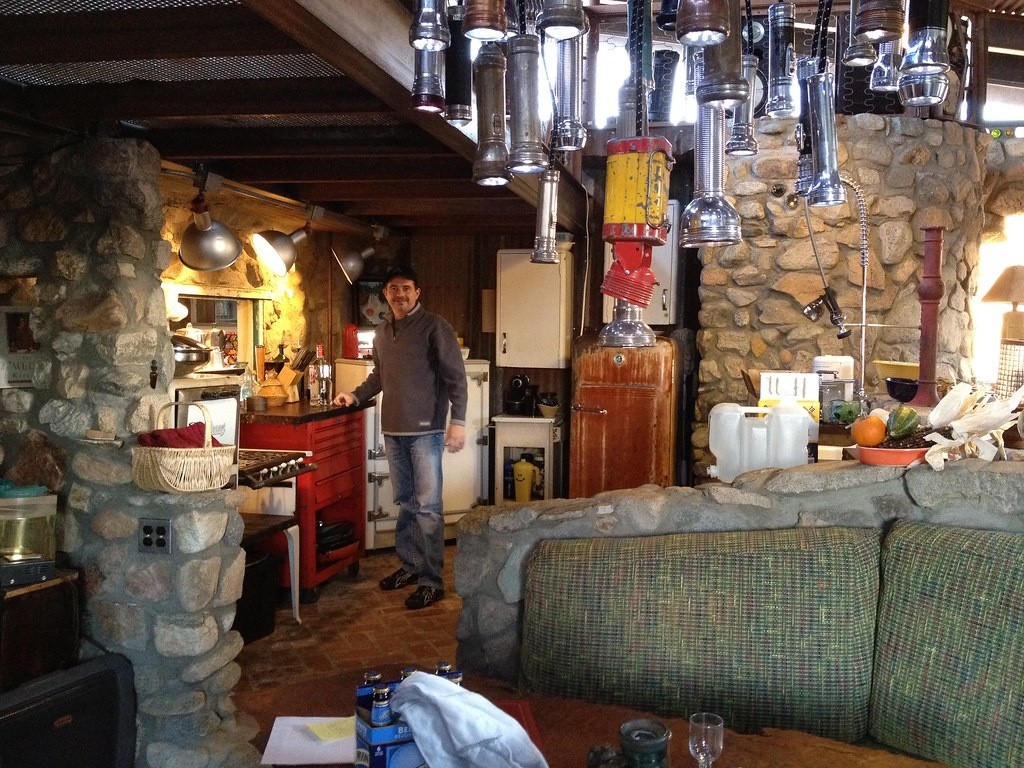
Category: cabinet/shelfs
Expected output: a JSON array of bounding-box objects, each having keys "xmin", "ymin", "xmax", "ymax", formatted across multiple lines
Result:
[
  {"xmin": 231, "ymin": 447, "xmax": 318, "ymax": 627},
  {"xmin": 494, "ymin": 249, "xmax": 575, "ymax": 369},
  {"xmin": 335, "ymin": 357, "xmax": 491, "ymax": 553},
  {"xmin": 491, "ymin": 414, "xmax": 567, "ymax": 507},
  {"xmin": 602, "ymin": 200, "xmax": 679, "ymax": 326},
  {"xmin": 570, "ymin": 332, "xmax": 675, "ymax": 501},
  {"xmin": 243, "ymin": 398, "xmax": 378, "ymax": 602}
]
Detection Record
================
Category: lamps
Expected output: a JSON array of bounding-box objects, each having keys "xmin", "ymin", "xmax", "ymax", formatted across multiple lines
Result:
[
  {"xmin": 331, "ymin": 221, "xmax": 391, "ymax": 287},
  {"xmin": 252, "ymin": 201, "xmax": 325, "ymax": 278},
  {"xmin": 981, "ymin": 264, "xmax": 1024, "ymax": 408},
  {"xmin": 158, "ymin": 168, "xmax": 242, "ymax": 272},
  {"xmin": 408, "ymin": 0, "xmax": 955, "ymax": 349}
]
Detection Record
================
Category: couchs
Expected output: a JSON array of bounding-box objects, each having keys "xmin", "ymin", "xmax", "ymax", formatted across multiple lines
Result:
[{"xmin": 456, "ymin": 524, "xmax": 1024, "ymax": 768}]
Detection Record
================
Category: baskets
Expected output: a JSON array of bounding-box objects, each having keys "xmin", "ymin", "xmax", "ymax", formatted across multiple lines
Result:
[{"xmin": 130, "ymin": 402, "xmax": 236, "ymax": 494}]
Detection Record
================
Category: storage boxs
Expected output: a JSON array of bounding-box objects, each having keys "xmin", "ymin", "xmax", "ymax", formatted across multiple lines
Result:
[
  {"xmin": 740, "ymin": 368, "xmax": 821, "ymax": 464},
  {"xmin": 354, "ymin": 682, "xmax": 426, "ymax": 768}
]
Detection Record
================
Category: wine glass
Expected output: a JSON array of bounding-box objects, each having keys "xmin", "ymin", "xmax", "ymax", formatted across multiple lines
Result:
[{"xmin": 689, "ymin": 712, "xmax": 724, "ymax": 768}]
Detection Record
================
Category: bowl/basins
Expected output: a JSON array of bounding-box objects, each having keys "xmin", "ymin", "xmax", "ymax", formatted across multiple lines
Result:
[
  {"xmin": 883, "ymin": 377, "xmax": 918, "ymax": 401},
  {"xmin": 856, "ymin": 445, "xmax": 929, "ymax": 467},
  {"xmin": 872, "ymin": 360, "xmax": 919, "ymax": 380}
]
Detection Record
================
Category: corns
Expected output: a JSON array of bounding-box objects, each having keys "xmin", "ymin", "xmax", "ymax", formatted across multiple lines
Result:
[{"xmin": 873, "ymin": 426, "xmax": 954, "ymax": 449}]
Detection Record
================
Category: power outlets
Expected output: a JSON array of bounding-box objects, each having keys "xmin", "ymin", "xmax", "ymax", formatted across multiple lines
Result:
[{"xmin": 137, "ymin": 517, "xmax": 173, "ymax": 555}]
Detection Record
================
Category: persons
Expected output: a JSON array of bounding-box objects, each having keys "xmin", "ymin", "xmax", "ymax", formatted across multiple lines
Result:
[{"xmin": 333, "ymin": 267, "xmax": 469, "ymax": 609}]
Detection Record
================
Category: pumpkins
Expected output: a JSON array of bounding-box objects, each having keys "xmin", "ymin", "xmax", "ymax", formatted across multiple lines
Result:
[
  {"xmin": 886, "ymin": 405, "xmax": 921, "ymax": 438},
  {"xmin": 851, "ymin": 412, "xmax": 886, "ymax": 445}
]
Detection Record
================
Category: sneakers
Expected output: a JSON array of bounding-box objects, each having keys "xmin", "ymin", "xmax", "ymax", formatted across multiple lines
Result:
[
  {"xmin": 380, "ymin": 568, "xmax": 419, "ymax": 590},
  {"xmin": 405, "ymin": 585, "xmax": 444, "ymax": 610}
]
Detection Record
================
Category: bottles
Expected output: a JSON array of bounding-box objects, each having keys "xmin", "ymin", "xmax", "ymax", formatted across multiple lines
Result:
[
  {"xmin": 273, "ymin": 344, "xmax": 290, "ymax": 366},
  {"xmin": 355, "ymin": 661, "xmax": 463, "ymax": 729},
  {"xmin": 309, "ymin": 345, "xmax": 333, "ymax": 407}
]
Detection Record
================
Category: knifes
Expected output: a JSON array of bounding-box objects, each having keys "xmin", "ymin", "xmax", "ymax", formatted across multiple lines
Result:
[{"xmin": 289, "ymin": 347, "xmax": 314, "ymax": 372}]
